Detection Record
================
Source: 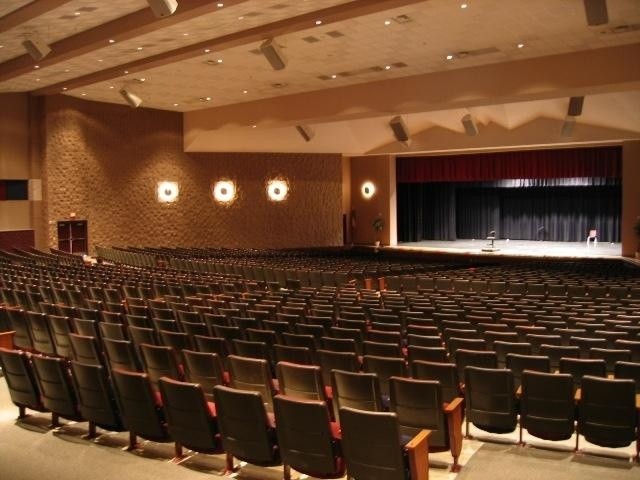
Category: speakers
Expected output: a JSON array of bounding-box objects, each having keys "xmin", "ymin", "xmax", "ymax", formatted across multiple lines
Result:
[
  {"xmin": 389, "ymin": 115, "xmax": 409, "ymax": 142},
  {"xmin": 147, "ymin": 0, "xmax": 178, "ymax": 19},
  {"xmin": 28, "ymin": 179, "xmax": 42, "ymax": 200},
  {"xmin": 583, "ymin": 0, "xmax": 608, "ymax": 26},
  {"xmin": 259, "ymin": 38, "xmax": 288, "ymax": 70},
  {"xmin": 567, "ymin": 97, "xmax": 584, "ymax": 116},
  {"xmin": 461, "ymin": 114, "xmax": 478, "ymax": 136},
  {"xmin": 23, "ymin": 33, "xmax": 52, "ymax": 63},
  {"xmin": 119, "ymin": 86, "xmax": 142, "ymax": 109},
  {"xmin": 296, "ymin": 123, "xmax": 313, "ymax": 142}
]
